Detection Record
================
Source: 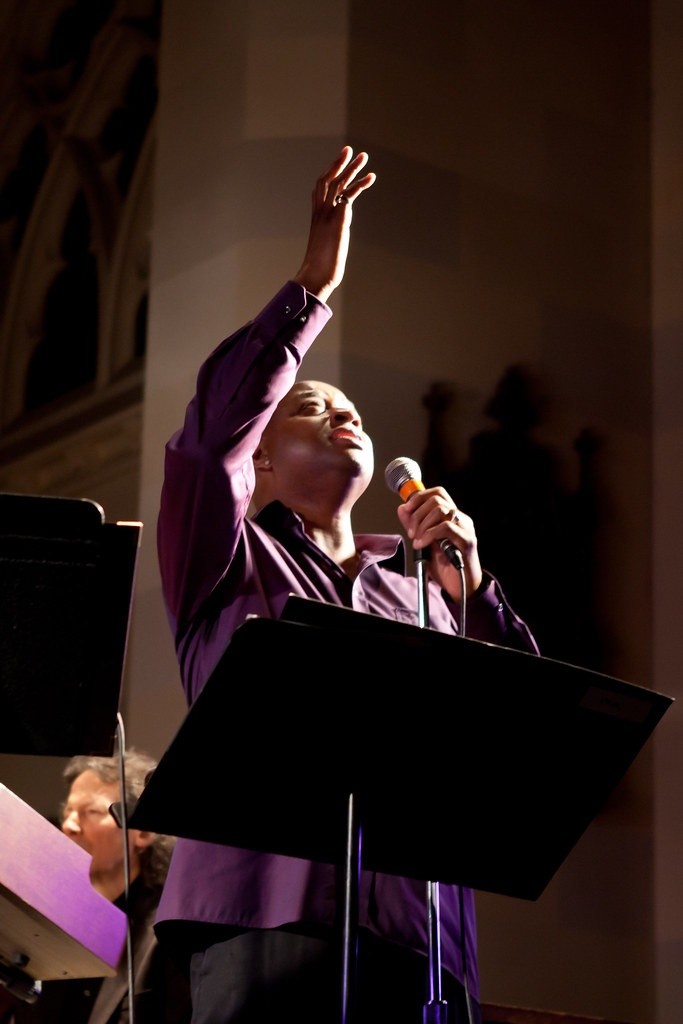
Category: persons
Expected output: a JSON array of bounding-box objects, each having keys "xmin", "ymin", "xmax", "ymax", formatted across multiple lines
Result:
[
  {"xmin": 152, "ymin": 144, "xmax": 541, "ymax": 1024},
  {"xmin": 20, "ymin": 747, "xmax": 192, "ymax": 1024}
]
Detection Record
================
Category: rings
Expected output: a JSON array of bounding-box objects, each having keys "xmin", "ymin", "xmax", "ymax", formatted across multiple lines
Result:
[{"xmin": 336, "ymin": 194, "xmax": 349, "ymax": 203}]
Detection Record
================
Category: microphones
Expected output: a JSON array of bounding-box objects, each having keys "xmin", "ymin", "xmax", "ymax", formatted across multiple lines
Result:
[{"xmin": 384, "ymin": 457, "xmax": 466, "ymax": 569}]
